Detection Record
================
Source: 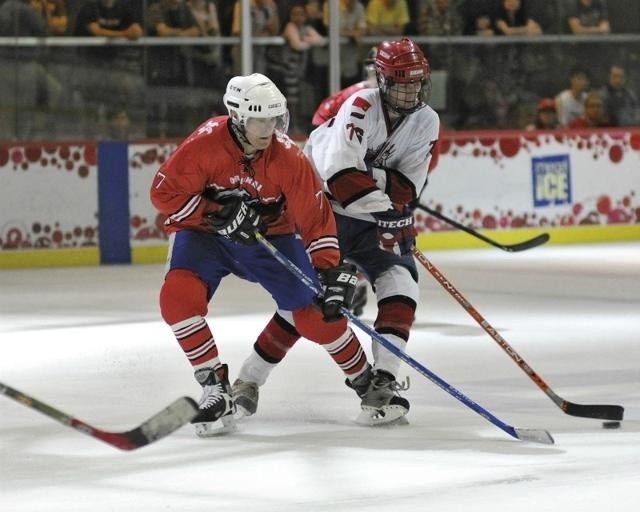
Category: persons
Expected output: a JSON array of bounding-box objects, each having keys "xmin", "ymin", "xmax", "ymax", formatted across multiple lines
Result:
[
  {"xmin": 310, "ymin": 45, "xmax": 440, "ymax": 319},
  {"xmin": 149, "ymin": 72, "xmax": 374, "ymax": 424},
  {"xmin": 229, "ymin": 36, "xmax": 440, "ymax": 412}
]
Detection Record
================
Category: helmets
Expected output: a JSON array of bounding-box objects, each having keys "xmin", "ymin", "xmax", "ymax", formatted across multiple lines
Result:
[
  {"xmin": 221, "ymin": 71, "xmax": 289, "ymax": 127},
  {"xmin": 373, "ymin": 36, "xmax": 432, "ymax": 89}
]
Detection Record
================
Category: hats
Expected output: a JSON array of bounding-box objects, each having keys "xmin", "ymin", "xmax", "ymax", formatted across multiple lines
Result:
[{"xmin": 538, "ymin": 97, "xmax": 557, "ymax": 114}]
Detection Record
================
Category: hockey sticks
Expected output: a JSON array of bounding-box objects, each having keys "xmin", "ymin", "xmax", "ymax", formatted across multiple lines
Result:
[
  {"xmin": 1, "ymin": 383, "xmax": 199, "ymax": 450},
  {"xmin": 411, "ymin": 247, "xmax": 625, "ymax": 422},
  {"xmin": 255, "ymin": 232, "xmax": 554, "ymax": 445},
  {"xmin": 417, "ymin": 204, "xmax": 550, "ymax": 253}
]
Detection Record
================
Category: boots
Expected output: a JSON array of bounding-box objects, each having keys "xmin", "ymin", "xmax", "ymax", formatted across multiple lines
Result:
[
  {"xmin": 225, "ymin": 376, "xmax": 260, "ymax": 418},
  {"xmin": 344, "ymin": 363, "xmax": 375, "ymax": 401},
  {"xmin": 185, "ymin": 363, "xmax": 241, "ymax": 425},
  {"xmin": 360, "ymin": 368, "xmax": 412, "ymax": 414}
]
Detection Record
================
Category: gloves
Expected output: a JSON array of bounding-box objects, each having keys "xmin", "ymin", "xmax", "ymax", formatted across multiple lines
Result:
[
  {"xmin": 370, "ymin": 210, "xmax": 419, "ymax": 257},
  {"xmin": 202, "ymin": 196, "xmax": 274, "ymax": 249},
  {"xmin": 311, "ymin": 262, "xmax": 368, "ymax": 324}
]
